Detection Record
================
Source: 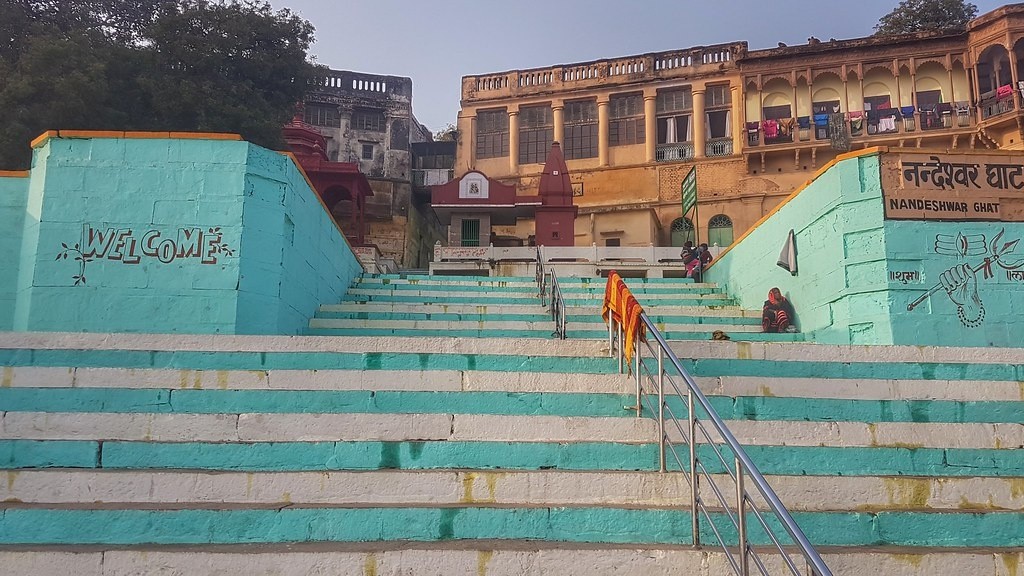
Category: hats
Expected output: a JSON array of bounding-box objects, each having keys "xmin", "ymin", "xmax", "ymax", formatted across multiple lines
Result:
[{"xmin": 684, "ymin": 241, "xmax": 692, "ymax": 248}]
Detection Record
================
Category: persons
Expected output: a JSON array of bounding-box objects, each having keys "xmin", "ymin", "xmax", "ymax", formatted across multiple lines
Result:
[
  {"xmin": 680, "ymin": 241, "xmax": 713, "ymax": 275},
  {"xmin": 762, "ymin": 287, "xmax": 794, "ymax": 333}
]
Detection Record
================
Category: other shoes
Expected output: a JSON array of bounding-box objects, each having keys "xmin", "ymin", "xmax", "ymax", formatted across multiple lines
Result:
[
  {"xmin": 687, "ymin": 274, "xmax": 694, "ymax": 278},
  {"xmin": 692, "ymin": 266, "xmax": 699, "ymax": 272}
]
